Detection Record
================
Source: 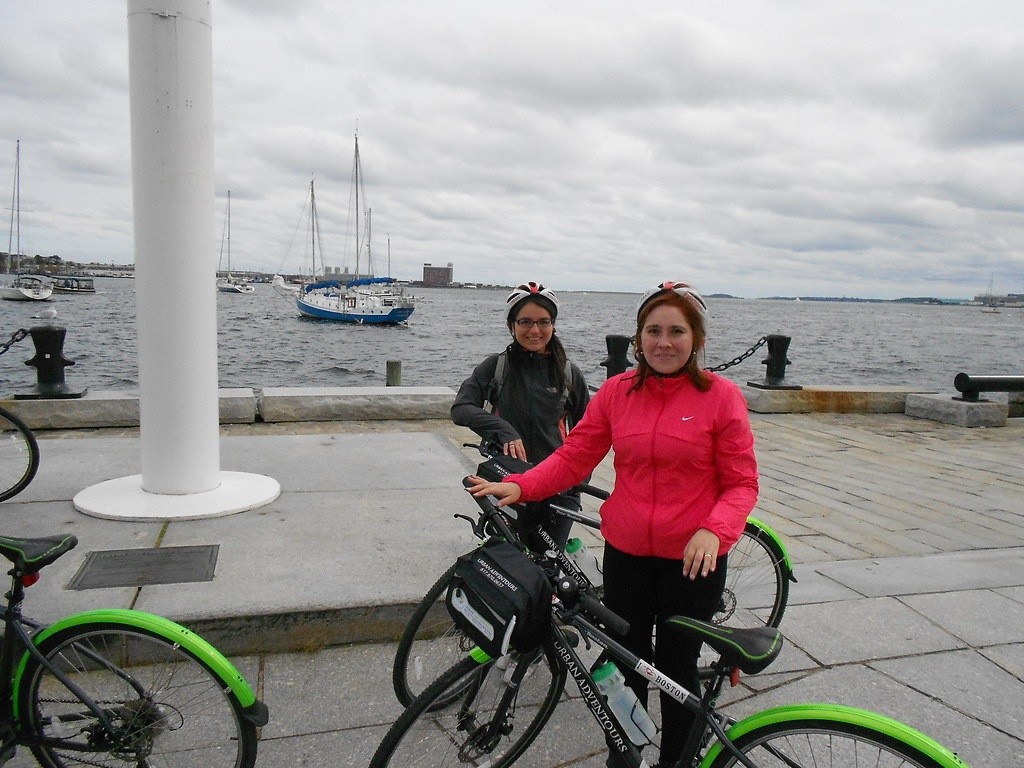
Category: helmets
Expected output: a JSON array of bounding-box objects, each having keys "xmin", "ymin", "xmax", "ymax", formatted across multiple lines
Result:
[
  {"xmin": 635, "ymin": 281, "xmax": 708, "ymax": 337},
  {"xmin": 504, "ymin": 281, "xmax": 561, "ymax": 318}
]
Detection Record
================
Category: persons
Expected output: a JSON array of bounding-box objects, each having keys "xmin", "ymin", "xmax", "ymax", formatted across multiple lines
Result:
[
  {"xmin": 451, "ymin": 283, "xmax": 591, "ymax": 684},
  {"xmin": 470, "ymin": 281, "xmax": 759, "ymax": 768}
]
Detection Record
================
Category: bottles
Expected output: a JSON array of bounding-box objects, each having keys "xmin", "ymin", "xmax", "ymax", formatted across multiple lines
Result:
[
  {"xmin": 565, "ymin": 536, "xmax": 604, "ymax": 587},
  {"xmin": 592, "ymin": 660, "xmax": 655, "ymax": 746}
]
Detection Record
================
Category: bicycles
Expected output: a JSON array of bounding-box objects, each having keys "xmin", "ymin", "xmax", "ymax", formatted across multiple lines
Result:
[
  {"xmin": 367, "ymin": 476, "xmax": 974, "ymax": 768},
  {"xmin": 0, "ymin": 532, "xmax": 270, "ymax": 768},
  {"xmin": 391, "ymin": 435, "xmax": 800, "ymax": 714}
]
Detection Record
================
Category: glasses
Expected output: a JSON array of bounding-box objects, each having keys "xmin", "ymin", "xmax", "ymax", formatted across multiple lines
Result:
[{"xmin": 511, "ymin": 318, "xmax": 556, "ymax": 327}]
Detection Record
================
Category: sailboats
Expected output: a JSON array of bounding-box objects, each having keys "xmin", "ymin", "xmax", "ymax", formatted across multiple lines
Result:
[
  {"xmin": 0, "ymin": 140, "xmax": 52, "ymax": 300},
  {"xmin": 216, "ymin": 190, "xmax": 254, "ymax": 293},
  {"xmin": 271, "ymin": 135, "xmax": 420, "ymax": 324}
]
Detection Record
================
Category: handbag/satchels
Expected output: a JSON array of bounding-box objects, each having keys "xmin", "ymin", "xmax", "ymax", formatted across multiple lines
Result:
[{"xmin": 446, "ymin": 534, "xmax": 555, "ymax": 660}]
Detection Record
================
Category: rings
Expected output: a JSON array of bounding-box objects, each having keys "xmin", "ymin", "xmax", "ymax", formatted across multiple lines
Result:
[
  {"xmin": 704, "ymin": 553, "xmax": 712, "ymax": 558},
  {"xmin": 508, "ymin": 444, "xmax": 514, "ymax": 448}
]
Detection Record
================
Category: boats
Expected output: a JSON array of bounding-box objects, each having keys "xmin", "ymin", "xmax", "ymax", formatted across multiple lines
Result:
[
  {"xmin": 982, "ymin": 307, "xmax": 1001, "ymax": 313},
  {"xmin": 48, "ymin": 274, "xmax": 96, "ymax": 296}
]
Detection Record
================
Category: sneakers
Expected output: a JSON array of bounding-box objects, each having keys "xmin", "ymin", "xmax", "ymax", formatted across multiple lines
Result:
[{"xmin": 496, "ymin": 651, "xmax": 540, "ymax": 686}]
